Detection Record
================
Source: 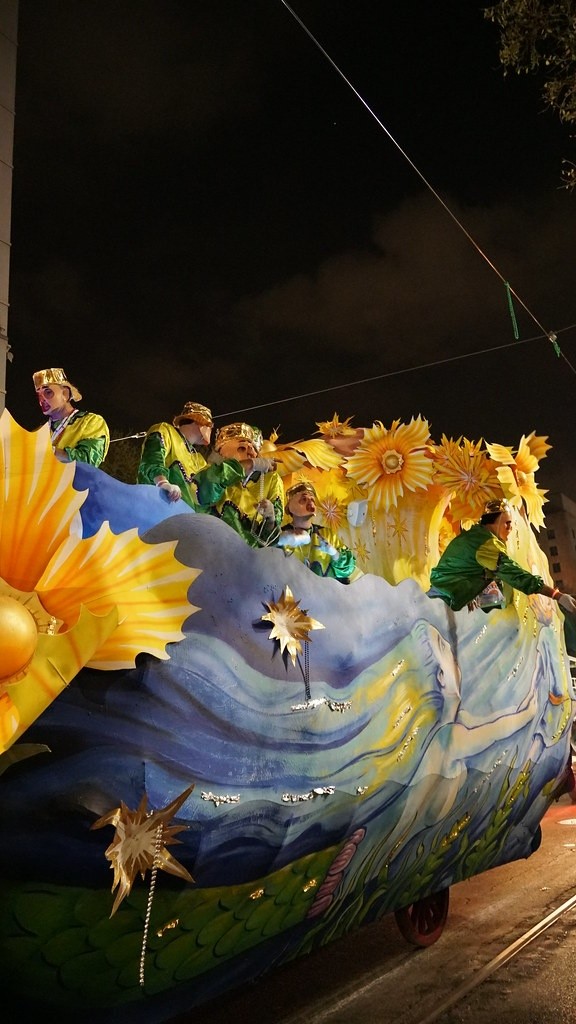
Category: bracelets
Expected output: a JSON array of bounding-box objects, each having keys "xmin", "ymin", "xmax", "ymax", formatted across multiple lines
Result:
[
  {"xmin": 52, "ymin": 446, "xmax": 55, "ymax": 455},
  {"xmin": 156, "ymin": 480, "xmax": 169, "ymax": 486},
  {"xmin": 552, "ymin": 589, "xmax": 559, "ymax": 600}
]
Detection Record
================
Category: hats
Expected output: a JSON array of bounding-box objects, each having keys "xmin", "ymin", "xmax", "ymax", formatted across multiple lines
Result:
[
  {"xmin": 215, "ymin": 422, "xmax": 262, "ymax": 452},
  {"xmin": 481, "ymin": 499, "xmax": 513, "ymax": 515},
  {"xmin": 284, "ymin": 482, "xmax": 316, "ymax": 515},
  {"xmin": 173, "ymin": 401, "xmax": 214, "ymax": 429},
  {"xmin": 32, "ymin": 367, "xmax": 82, "ymax": 402}
]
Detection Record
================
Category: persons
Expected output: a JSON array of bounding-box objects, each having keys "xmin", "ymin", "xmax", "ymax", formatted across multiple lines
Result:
[
  {"xmin": 425, "ymin": 498, "xmax": 576, "ymax": 613},
  {"xmin": 33, "ymin": 367, "xmax": 110, "ymax": 468},
  {"xmin": 268, "ymin": 482, "xmax": 356, "ymax": 579},
  {"xmin": 195, "ymin": 422, "xmax": 285, "ymax": 549},
  {"xmin": 136, "ymin": 401, "xmax": 213, "ymax": 514}
]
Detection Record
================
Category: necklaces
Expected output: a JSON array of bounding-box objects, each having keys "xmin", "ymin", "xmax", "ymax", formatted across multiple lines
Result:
[{"xmin": 292, "ymin": 523, "xmax": 315, "ymax": 568}]
